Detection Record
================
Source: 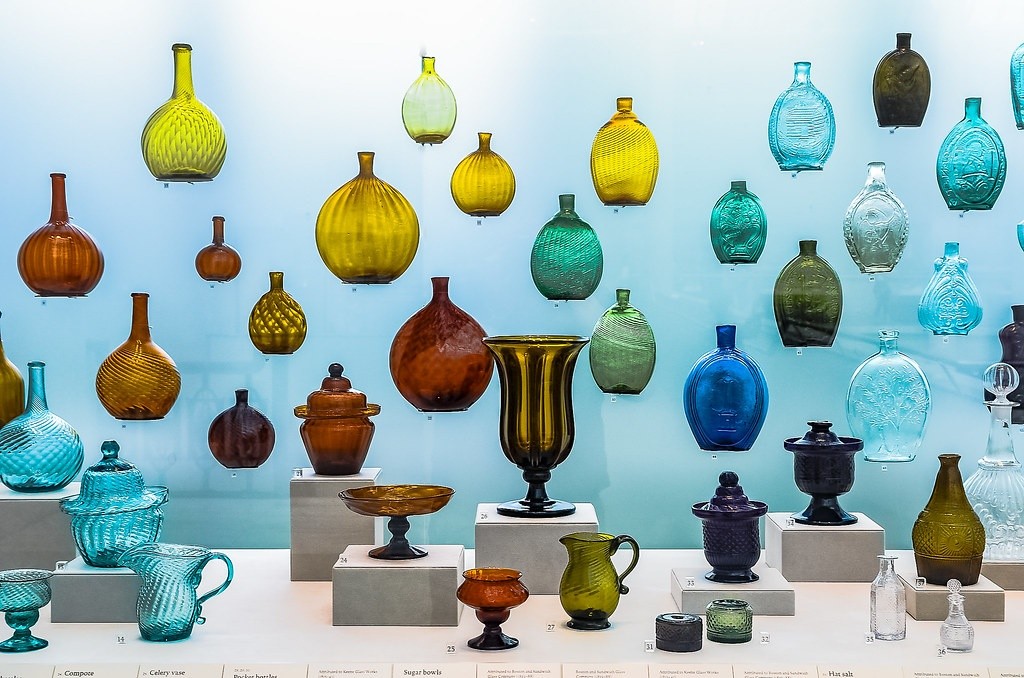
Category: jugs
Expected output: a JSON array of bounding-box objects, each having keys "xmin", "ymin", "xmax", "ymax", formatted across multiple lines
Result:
[
  {"xmin": 558, "ymin": 532, "xmax": 640, "ymax": 632},
  {"xmin": 116, "ymin": 542, "xmax": 235, "ymax": 641}
]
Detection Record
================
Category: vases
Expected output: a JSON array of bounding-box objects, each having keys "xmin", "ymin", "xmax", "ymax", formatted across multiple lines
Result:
[{"xmin": 0, "ymin": 34, "xmax": 1024, "ymax": 653}]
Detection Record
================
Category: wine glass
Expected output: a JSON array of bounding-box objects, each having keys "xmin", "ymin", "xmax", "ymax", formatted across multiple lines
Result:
[
  {"xmin": 0, "ymin": 569, "xmax": 54, "ymax": 652},
  {"xmin": 482, "ymin": 334, "xmax": 590, "ymax": 517}
]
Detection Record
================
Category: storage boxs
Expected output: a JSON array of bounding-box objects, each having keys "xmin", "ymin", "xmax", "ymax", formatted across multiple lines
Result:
[
  {"xmin": 332, "ymin": 546, "xmax": 465, "ymax": 628},
  {"xmin": 50, "ymin": 555, "xmax": 142, "ymax": 623},
  {"xmin": 672, "ymin": 569, "xmax": 795, "ymax": 616},
  {"xmin": 290, "ymin": 467, "xmax": 385, "ymax": 582},
  {"xmin": 981, "ymin": 561, "xmax": 1024, "ymax": 591},
  {"xmin": 764, "ymin": 511, "xmax": 885, "ymax": 583},
  {"xmin": 898, "ymin": 573, "xmax": 1005, "ymax": 622},
  {"xmin": 475, "ymin": 502, "xmax": 599, "ymax": 595},
  {"xmin": 0, "ymin": 481, "xmax": 81, "ymax": 571}
]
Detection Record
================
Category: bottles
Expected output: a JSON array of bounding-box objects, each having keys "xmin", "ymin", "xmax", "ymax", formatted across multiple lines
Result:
[{"xmin": 0, "ymin": 32, "xmax": 1023, "ymax": 653}]
